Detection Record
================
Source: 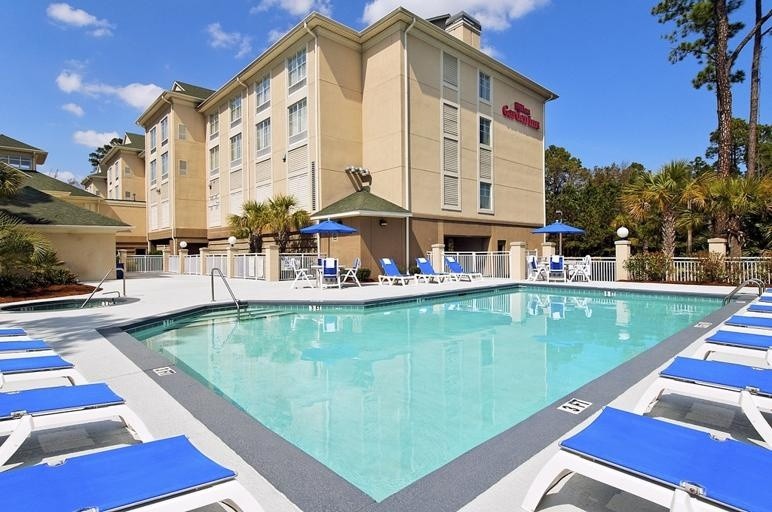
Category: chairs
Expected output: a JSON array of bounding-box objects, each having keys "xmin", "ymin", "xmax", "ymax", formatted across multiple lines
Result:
[
  {"xmin": 525, "ymin": 253, "xmax": 592, "ymax": 284},
  {"xmin": 291, "ymin": 256, "xmax": 363, "ymax": 290},
  {"xmin": 377, "ymin": 257, "xmax": 484, "ymax": 286}
]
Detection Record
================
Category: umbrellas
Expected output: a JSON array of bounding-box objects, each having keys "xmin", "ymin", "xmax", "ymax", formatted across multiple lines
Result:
[
  {"xmin": 300, "ymin": 216, "xmax": 361, "ymax": 258},
  {"xmin": 529, "ymin": 220, "xmax": 585, "ymax": 255}
]
